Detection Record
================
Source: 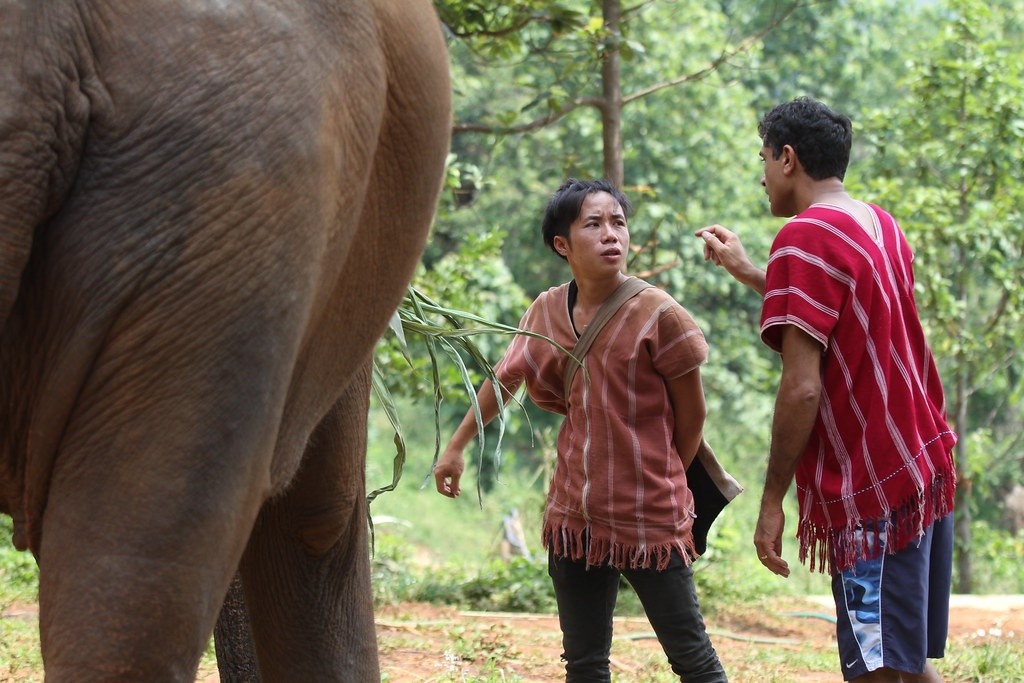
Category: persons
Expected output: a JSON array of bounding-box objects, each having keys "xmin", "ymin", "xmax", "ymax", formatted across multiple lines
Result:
[
  {"xmin": 696, "ymin": 95, "xmax": 959, "ymax": 683},
  {"xmin": 433, "ymin": 175, "xmax": 744, "ymax": 683}
]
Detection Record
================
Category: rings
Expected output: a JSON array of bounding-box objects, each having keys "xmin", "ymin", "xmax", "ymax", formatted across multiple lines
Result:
[{"xmin": 760, "ymin": 554, "xmax": 768, "ymax": 560}]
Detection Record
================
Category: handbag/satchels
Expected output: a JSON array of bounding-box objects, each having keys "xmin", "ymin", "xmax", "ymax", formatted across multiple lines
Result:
[{"xmin": 685, "ymin": 438, "xmax": 745, "ymax": 562}]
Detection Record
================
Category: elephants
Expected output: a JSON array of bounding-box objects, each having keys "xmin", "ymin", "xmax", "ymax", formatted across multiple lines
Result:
[{"xmin": 2, "ymin": 0, "xmax": 453, "ymax": 683}]
